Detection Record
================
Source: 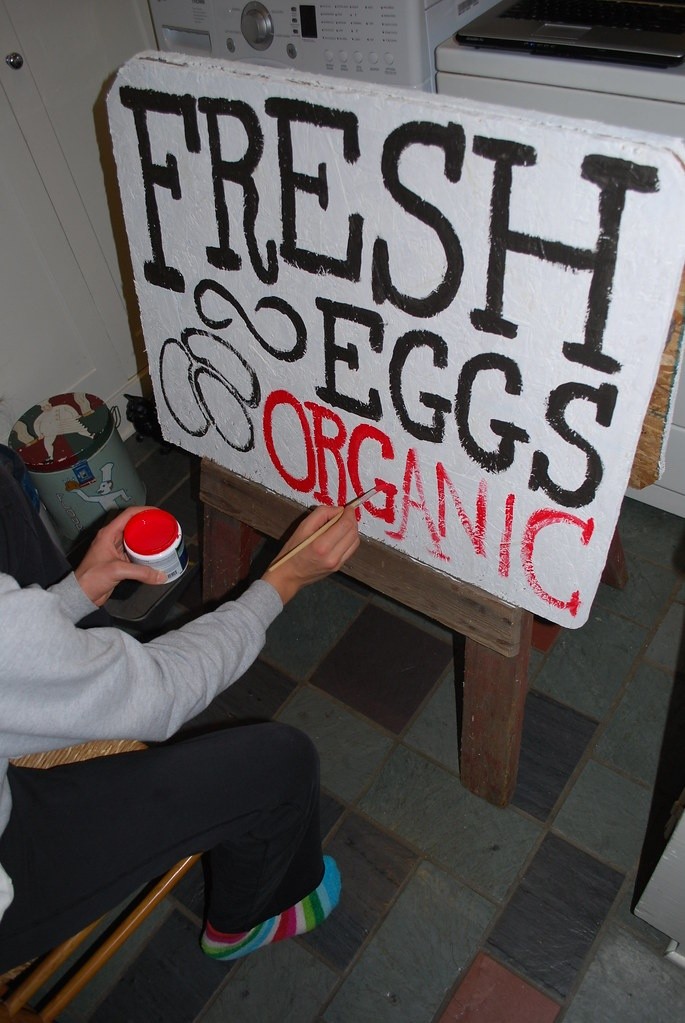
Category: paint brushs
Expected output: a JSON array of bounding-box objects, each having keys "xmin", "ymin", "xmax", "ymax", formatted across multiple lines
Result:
[{"xmin": 267, "ymin": 482, "xmax": 388, "ymax": 576}]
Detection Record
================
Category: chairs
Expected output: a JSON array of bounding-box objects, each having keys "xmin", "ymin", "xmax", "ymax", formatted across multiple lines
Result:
[{"xmin": 0, "ymin": 734, "xmax": 198, "ymax": 1022}]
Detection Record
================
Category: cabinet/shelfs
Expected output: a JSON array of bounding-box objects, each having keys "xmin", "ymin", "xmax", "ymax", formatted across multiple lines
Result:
[{"xmin": 436, "ymin": 51, "xmax": 684, "ymax": 521}]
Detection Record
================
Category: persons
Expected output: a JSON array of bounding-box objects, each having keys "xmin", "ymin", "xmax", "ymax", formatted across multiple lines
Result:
[{"xmin": 0, "ymin": 501, "xmax": 363, "ymax": 981}]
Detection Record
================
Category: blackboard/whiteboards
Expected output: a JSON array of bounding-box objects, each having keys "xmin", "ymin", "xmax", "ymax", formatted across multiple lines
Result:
[{"xmin": 88, "ymin": 51, "xmax": 684, "ymax": 658}]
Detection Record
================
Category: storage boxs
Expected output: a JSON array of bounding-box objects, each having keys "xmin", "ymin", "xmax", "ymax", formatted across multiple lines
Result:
[{"xmin": 7, "ymin": 393, "xmax": 151, "ymax": 543}]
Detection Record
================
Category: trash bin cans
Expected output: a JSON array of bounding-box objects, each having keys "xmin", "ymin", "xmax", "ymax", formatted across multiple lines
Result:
[{"xmin": 7, "ymin": 394, "xmax": 149, "ymax": 543}]
